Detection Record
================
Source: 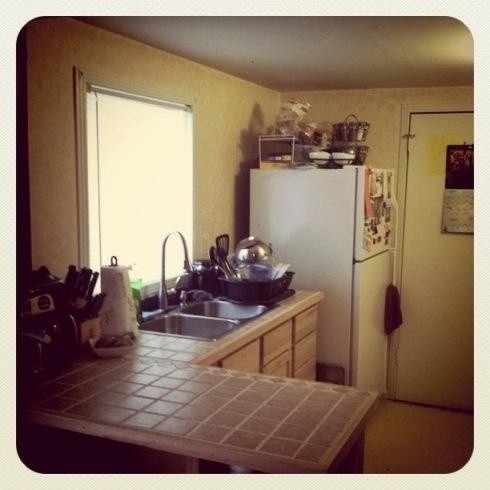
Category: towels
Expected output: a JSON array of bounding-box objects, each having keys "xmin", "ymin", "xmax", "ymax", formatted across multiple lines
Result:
[{"xmin": 384, "ymin": 283, "xmax": 402, "ymax": 336}]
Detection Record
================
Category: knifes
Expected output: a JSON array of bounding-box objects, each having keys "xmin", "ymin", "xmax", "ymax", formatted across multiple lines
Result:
[{"xmin": 62, "ymin": 263, "xmax": 107, "ymax": 322}]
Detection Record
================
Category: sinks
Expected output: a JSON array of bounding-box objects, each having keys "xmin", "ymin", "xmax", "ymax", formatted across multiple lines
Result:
[
  {"xmin": 137, "ymin": 309, "xmax": 240, "ymax": 341},
  {"xmin": 176, "ymin": 298, "xmax": 279, "ymax": 320}
]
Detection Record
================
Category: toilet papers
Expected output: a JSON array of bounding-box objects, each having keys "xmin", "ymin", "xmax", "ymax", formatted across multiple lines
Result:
[{"xmin": 98, "ymin": 264, "xmax": 139, "ymax": 339}]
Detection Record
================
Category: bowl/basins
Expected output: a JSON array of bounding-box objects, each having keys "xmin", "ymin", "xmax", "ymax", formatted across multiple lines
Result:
[
  {"xmin": 236, "ymin": 236, "xmax": 274, "ymax": 267},
  {"xmin": 268, "ymin": 152, "xmax": 292, "ymax": 161},
  {"xmin": 308, "ymin": 151, "xmax": 354, "ymax": 164},
  {"xmin": 89, "ymin": 337, "xmax": 136, "ymax": 358},
  {"xmin": 332, "ymin": 121, "xmax": 371, "ymax": 143},
  {"xmin": 290, "ymin": 143, "xmax": 320, "ymax": 163}
]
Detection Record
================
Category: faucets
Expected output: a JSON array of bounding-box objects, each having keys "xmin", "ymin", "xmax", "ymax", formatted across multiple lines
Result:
[{"xmin": 157, "ymin": 228, "xmax": 191, "ymax": 311}]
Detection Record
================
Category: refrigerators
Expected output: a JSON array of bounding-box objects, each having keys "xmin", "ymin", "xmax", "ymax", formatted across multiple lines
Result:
[{"xmin": 249, "ymin": 167, "xmax": 397, "ymax": 395}]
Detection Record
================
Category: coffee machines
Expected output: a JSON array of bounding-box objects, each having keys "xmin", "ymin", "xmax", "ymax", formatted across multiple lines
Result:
[{"xmin": 16, "ymin": 264, "xmax": 80, "ymax": 383}]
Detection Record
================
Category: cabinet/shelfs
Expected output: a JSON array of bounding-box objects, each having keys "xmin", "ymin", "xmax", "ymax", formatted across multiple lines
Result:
[
  {"xmin": 292, "ymin": 299, "xmax": 319, "ymax": 383},
  {"xmin": 261, "ymin": 314, "xmax": 293, "ymax": 380},
  {"xmin": 257, "ymin": 132, "xmax": 314, "ymax": 170},
  {"xmin": 218, "ymin": 337, "xmax": 262, "ymax": 372}
]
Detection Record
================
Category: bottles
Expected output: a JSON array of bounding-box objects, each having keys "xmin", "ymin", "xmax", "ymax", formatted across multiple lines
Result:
[{"xmin": 134, "ymin": 289, "xmax": 143, "ymax": 325}]
